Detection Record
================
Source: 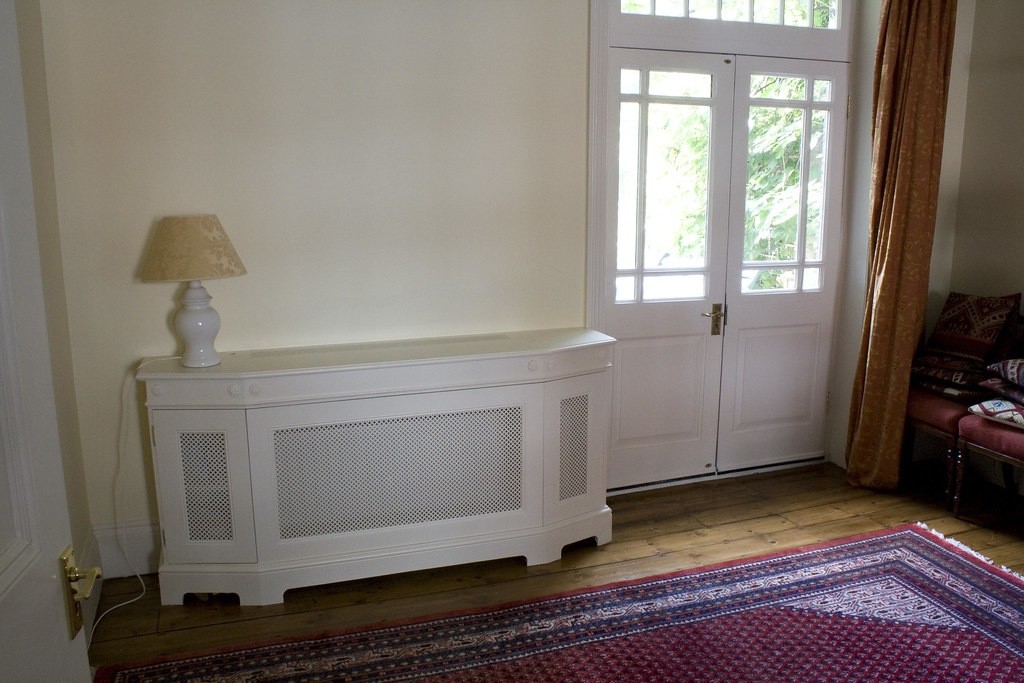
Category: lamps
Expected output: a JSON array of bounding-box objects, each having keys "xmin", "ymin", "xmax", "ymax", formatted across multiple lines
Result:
[{"xmin": 140, "ymin": 214, "xmax": 248, "ymax": 369}]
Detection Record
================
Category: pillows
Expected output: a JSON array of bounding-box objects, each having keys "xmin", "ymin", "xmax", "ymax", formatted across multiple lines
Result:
[{"xmin": 910, "ymin": 292, "xmax": 1024, "ymax": 431}]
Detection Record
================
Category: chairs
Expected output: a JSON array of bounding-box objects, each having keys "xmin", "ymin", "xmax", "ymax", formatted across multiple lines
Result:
[
  {"xmin": 953, "ymin": 321, "xmax": 1024, "ymax": 519},
  {"xmin": 904, "ymin": 290, "xmax": 1021, "ymax": 511}
]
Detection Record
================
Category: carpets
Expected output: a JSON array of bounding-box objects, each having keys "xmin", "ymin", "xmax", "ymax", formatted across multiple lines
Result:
[{"xmin": 91, "ymin": 521, "xmax": 1024, "ymax": 683}]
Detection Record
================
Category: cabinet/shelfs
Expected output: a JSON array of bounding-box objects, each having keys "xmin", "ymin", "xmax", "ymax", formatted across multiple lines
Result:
[{"xmin": 134, "ymin": 327, "xmax": 618, "ymax": 606}]
